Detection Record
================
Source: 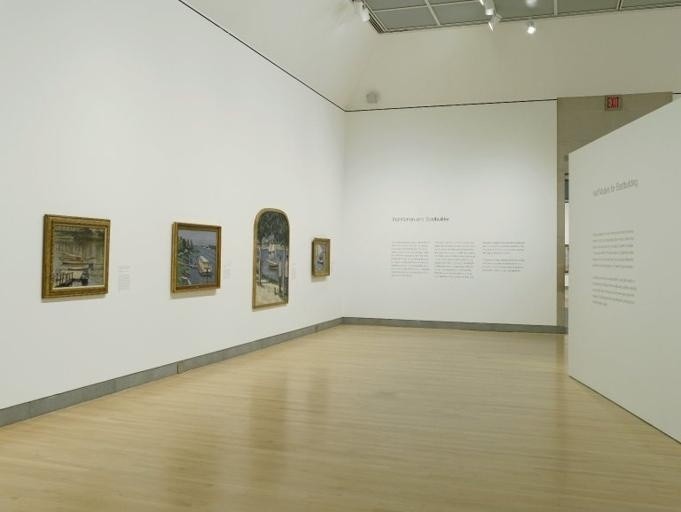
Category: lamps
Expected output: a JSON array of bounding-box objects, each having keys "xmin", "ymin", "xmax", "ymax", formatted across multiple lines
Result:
[
  {"xmin": 526, "ymin": 19, "xmax": 535, "ymax": 34},
  {"xmin": 480, "ymin": 0, "xmax": 502, "ymax": 32}
]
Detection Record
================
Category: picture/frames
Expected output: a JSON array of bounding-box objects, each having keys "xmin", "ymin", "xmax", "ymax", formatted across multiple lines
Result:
[
  {"xmin": 41, "ymin": 213, "xmax": 110, "ymax": 298},
  {"xmin": 312, "ymin": 237, "xmax": 331, "ymax": 277},
  {"xmin": 171, "ymin": 221, "xmax": 222, "ymax": 294}
]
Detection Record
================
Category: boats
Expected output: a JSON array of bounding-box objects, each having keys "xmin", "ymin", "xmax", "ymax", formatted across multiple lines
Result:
[{"xmin": 196, "ymin": 256, "xmax": 213, "ymax": 277}]
